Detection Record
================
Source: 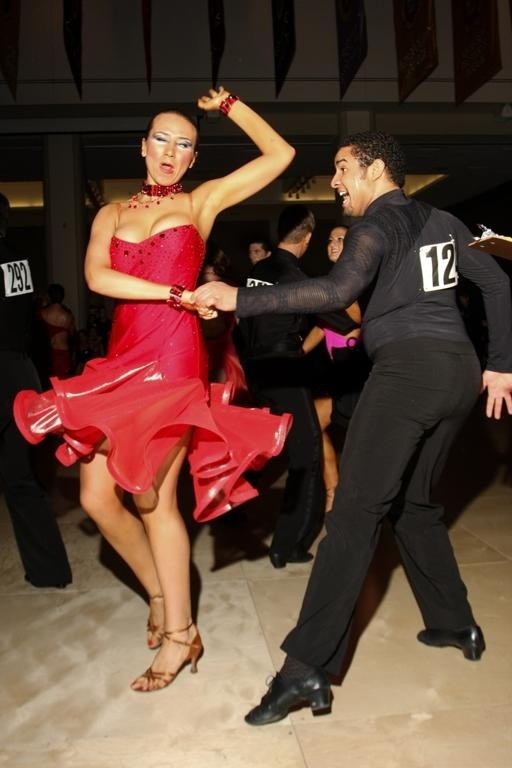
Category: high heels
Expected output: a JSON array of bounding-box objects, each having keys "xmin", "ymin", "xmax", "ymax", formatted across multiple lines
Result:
[
  {"xmin": 129, "ymin": 620, "xmax": 204, "ymax": 692},
  {"xmin": 143, "ymin": 588, "xmax": 165, "ymax": 651}
]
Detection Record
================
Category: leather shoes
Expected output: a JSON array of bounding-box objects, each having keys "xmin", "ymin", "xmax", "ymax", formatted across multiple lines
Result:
[
  {"xmin": 415, "ymin": 622, "xmax": 486, "ymax": 660},
  {"xmin": 241, "ymin": 669, "xmax": 334, "ymax": 726},
  {"xmin": 267, "ymin": 546, "xmax": 315, "ymax": 569}
]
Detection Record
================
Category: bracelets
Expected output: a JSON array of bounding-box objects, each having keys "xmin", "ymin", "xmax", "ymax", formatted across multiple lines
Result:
[{"xmin": 218, "ymin": 93, "xmax": 240, "ymax": 120}]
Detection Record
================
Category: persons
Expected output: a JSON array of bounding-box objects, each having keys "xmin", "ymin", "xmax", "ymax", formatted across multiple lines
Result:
[
  {"xmin": 186, "ymin": 129, "xmax": 512, "ymax": 725},
  {"xmin": 12, "ymin": 86, "xmax": 296, "ymax": 692}
]
